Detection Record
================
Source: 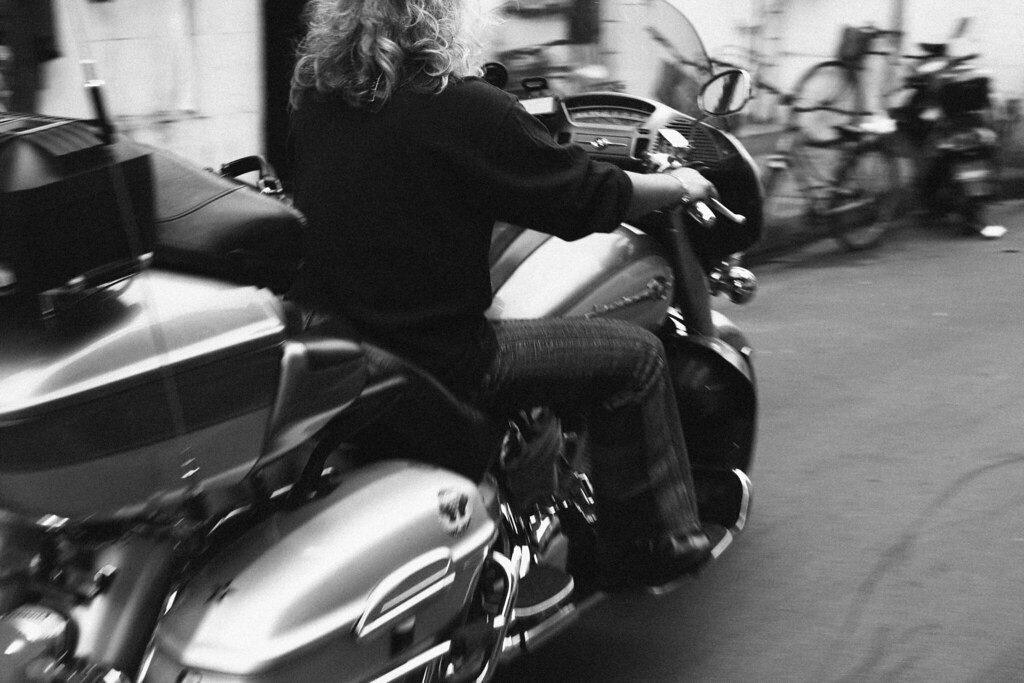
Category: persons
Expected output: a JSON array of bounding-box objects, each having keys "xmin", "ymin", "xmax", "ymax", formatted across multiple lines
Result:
[{"xmin": 282, "ymin": 0, "xmax": 719, "ymax": 598}]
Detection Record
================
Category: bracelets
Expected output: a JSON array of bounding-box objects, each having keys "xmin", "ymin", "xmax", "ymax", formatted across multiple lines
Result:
[{"xmin": 662, "ymin": 173, "xmax": 692, "ymax": 216}]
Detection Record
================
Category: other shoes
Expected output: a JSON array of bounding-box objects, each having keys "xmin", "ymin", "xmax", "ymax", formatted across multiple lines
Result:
[{"xmin": 588, "ymin": 528, "xmax": 709, "ymax": 594}]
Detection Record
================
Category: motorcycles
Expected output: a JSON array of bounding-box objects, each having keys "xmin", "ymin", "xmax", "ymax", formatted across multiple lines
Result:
[{"xmin": 0, "ymin": 1, "xmax": 766, "ymax": 677}]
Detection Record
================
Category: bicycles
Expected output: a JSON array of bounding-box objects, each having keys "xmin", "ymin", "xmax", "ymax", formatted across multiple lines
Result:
[{"xmin": 712, "ymin": 58, "xmax": 906, "ymax": 256}]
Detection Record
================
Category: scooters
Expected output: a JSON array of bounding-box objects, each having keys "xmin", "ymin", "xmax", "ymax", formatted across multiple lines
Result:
[{"xmin": 877, "ymin": 18, "xmax": 1002, "ymax": 238}]
